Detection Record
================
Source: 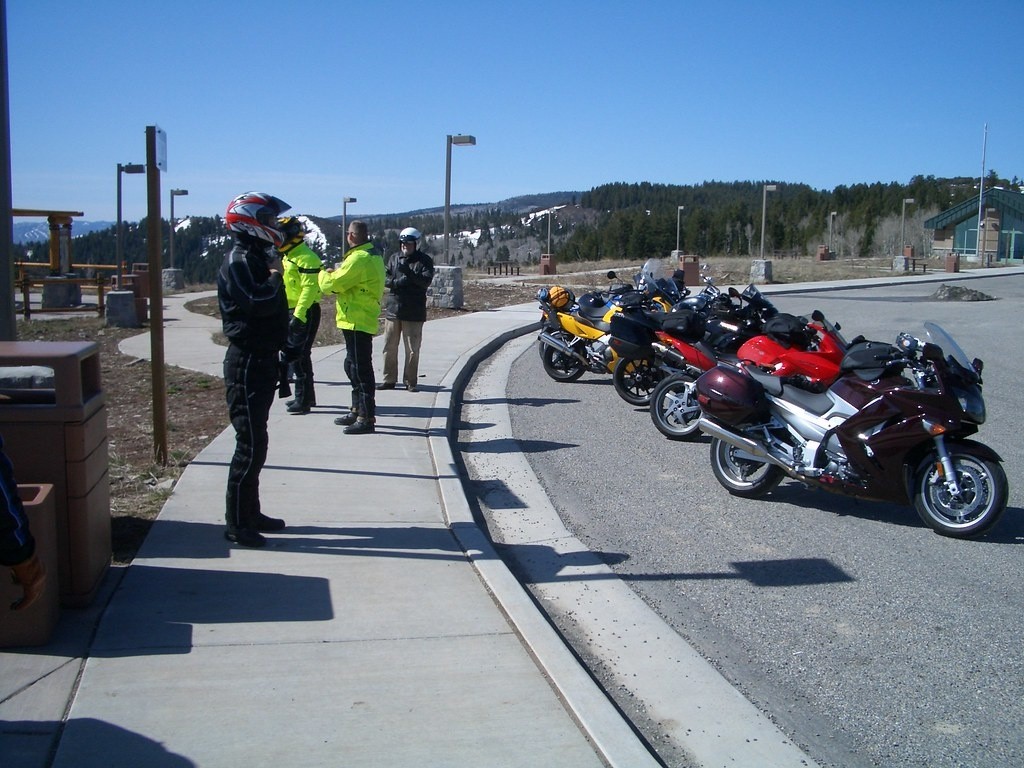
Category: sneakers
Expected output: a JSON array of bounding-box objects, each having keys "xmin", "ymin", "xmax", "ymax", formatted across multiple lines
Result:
[
  {"xmin": 252, "ymin": 512, "xmax": 285, "ymax": 531},
  {"xmin": 226, "ymin": 526, "xmax": 266, "ymax": 548}
]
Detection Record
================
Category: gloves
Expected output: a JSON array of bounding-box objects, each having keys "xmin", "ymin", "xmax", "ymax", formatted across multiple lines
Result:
[
  {"xmin": 287, "ymin": 315, "xmax": 299, "ymax": 333},
  {"xmin": 393, "ymin": 276, "xmax": 408, "ymax": 289},
  {"xmin": 396, "ymin": 260, "xmax": 411, "ymax": 275}
]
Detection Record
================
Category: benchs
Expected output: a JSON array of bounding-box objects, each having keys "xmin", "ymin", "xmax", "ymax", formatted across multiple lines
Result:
[
  {"xmin": 487, "ymin": 266, "xmax": 498, "ymax": 275},
  {"xmin": 510, "ymin": 266, "xmax": 521, "ymax": 275},
  {"xmin": 911, "ymin": 263, "xmax": 928, "ymax": 273}
]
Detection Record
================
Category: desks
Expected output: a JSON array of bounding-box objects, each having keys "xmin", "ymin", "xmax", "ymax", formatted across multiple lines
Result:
[
  {"xmin": 495, "ymin": 261, "xmax": 514, "ymax": 275},
  {"xmin": 908, "ymin": 258, "xmax": 923, "ymax": 272},
  {"xmin": 769, "ymin": 250, "xmax": 797, "ymax": 261}
]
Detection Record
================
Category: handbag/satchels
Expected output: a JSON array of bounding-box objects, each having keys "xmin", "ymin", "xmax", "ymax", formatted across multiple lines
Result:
[{"xmin": 763, "ymin": 313, "xmax": 810, "ymax": 349}]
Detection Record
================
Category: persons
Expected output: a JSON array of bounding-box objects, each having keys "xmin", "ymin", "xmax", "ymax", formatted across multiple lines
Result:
[
  {"xmin": 377, "ymin": 228, "xmax": 433, "ymax": 392},
  {"xmin": 319, "ymin": 220, "xmax": 386, "ymax": 434},
  {"xmin": 278, "ymin": 216, "xmax": 323, "ymax": 415},
  {"xmin": 217, "ymin": 191, "xmax": 292, "ymax": 547}
]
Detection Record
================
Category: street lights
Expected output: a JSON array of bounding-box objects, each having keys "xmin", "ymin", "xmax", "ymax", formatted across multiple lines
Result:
[
  {"xmin": 828, "ymin": 211, "xmax": 837, "ymax": 260},
  {"xmin": 334, "ymin": 197, "xmax": 357, "ymax": 270},
  {"xmin": 547, "ymin": 208, "xmax": 556, "ymax": 255},
  {"xmin": 161, "ymin": 188, "xmax": 189, "ymax": 290},
  {"xmin": 671, "ymin": 205, "xmax": 684, "ymax": 262},
  {"xmin": 750, "ymin": 184, "xmax": 777, "ymax": 284},
  {"xmin": 425, "ymin": 134, "xmax": 476, "ymax": 308},
  {"xmin": 98, "ymin": 162, "xmax": 145, "ymax": 327},
  {"xmin": 892, "ymin": 198, "xmax": 915, "ymax": 272}
]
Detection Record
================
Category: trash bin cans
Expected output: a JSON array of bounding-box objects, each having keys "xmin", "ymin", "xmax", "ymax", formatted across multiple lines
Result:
[
  {"xmin": 540, "ymin": 254, "xmax": 556, "ymax": 275},
  {"xmin": 904, "ymin": 245, "xmax": 914, "ymax": 257},
  {"xmin": 817, "ymin": 245, "xmax": 829, "ymax": 261},
  {"xmin": 540, "ymin": 264, "xmax": 548, "ymax": 275},
  {"xmin": 945, "ymin": 253, "xmax": 960, "ymax": 272},
  {"xmin": 679, "ymin": 254, "xmax": 699, "ymax": 286},
  {"xmin": 110, "ymin": 274, "xmax": 147, "ymax": 327},
  {"xmin": 132, "ymin": 263, "xmax": 149, "ymax": 298},
  {"xmin": 0, "ymin": 483, "xmax": 58, "ymax": 647},
  {"xmin": 0, "ymin": 336, "xmax": 114, "ymax": 615}
]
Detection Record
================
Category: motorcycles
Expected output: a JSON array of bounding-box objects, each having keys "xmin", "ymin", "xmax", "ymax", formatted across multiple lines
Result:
[{"xmin": 535, "ymin": 258, "xmax": 1009, "ymax": 539}]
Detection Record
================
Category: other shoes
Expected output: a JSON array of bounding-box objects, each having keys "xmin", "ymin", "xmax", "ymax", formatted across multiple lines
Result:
[
  {"xmin": 334, "ymin": 413, "xmax": 358, "ymax": 425},
  {"xmin": 287, "ymin": 400, "xmax": 310, "ymax": 413},
  {"xmin": 343, "ymin": 416, "xmax": 375, "ymax": 433},
  {"xmin": 374, "ymin": 381, "xmax": 395, "ymax": 389},
  {"xmin": 285, "ymin": 398, "xmax": 315, "ymax": 406},
  {"xmin": 407, "ymin": 385, "xmax": 418, "ymax": 392}
]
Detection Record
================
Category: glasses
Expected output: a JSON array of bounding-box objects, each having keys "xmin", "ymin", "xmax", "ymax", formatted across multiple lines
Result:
[{"xmin": 345, "ymin": 231, "xmax": 355, "ymax": 236}]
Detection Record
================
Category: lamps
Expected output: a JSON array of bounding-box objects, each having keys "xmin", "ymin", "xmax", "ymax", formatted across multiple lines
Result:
[
  {"xmin": 980, "ymin": 223, "xmax": 984, "ymax": 228},
  {"xmin": 992, "ymin": 223, "xmax": 997, "ymax": 227}
]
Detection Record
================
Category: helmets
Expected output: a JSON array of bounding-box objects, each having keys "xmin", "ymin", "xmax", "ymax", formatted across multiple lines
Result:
[
  {"xmin": 226, "ymin": 190, "xmax": 285, "ymax": 250},
  {"xmin": 272, "ymin": 215, "xmax": 304, "ymax": 252},
  {"xmin": 399, "ymin": 228, "xmax": 421, "ymax": 252}
]
